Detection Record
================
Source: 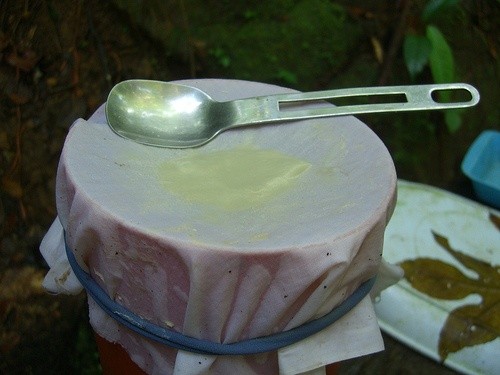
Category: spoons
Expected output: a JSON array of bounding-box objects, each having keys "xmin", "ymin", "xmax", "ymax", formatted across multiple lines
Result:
[{"xmin": 106, "ymin": 79, "xmax": 480, "ymax": 149}]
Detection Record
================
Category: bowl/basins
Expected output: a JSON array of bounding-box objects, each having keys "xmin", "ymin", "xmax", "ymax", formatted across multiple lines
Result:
[{"xmin": 461, "ymin": 131, "xmax": 500, "ymax": 208}]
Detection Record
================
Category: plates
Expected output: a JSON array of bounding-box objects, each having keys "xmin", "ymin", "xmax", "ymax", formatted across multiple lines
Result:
[{"xmin": 369, "ymin": 178, "xmax": 499, "ymax": 375}]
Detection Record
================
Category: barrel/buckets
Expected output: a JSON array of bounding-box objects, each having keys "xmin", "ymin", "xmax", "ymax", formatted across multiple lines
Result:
[{"xmin": 54, "ymin": 79, "xmax": 398, "ymax": 375}]
